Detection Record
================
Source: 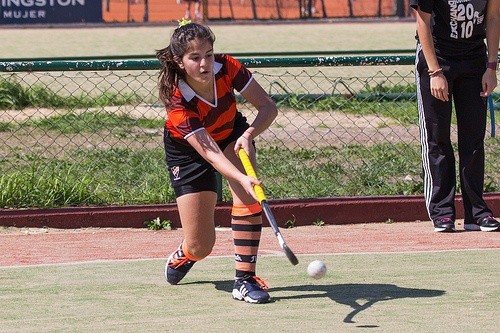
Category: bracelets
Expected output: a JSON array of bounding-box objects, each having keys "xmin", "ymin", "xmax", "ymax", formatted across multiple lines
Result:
[
  {"xmin": 428, "ymin": 68, "xmax": 442, "ymax": 77},
  {"xmin": 486, "ymin": 63, "xmax": 496, "ymax": 70}
]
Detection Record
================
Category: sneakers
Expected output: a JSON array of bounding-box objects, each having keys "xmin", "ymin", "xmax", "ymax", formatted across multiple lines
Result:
[
  {"xmin": 464, "ymin": 215, "xmax": 500, "ymax": 231},
  {"xmin": 165, "ymin": 239, "xmax": 197, "ymax": 286},
  {"xmin": 433, "ymin": 217, "xmax": 456, "ymax": 232},
  {"xmin": 232, "ymin": 275, "xmax": 270, "ymax": 304}
]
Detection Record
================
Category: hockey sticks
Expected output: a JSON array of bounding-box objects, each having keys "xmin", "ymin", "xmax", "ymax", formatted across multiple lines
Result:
[{"xmin": 240, "ymin": 149, "xmax": 299, "ymax": 267}]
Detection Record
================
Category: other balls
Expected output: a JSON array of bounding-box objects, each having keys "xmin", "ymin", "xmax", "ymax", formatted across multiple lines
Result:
[{"xmin": 307, "ymin": 259, "xmax": 328, "ymax": 279}]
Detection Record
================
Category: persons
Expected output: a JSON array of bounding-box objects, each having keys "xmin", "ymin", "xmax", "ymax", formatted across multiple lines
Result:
[
  {"xmin": 410, "ymin": 0, "xmax": 500, "ymax": 232},
  {"xmin": 155, "ymin": 23, "xmax": 278, "ymax": 304}
]
